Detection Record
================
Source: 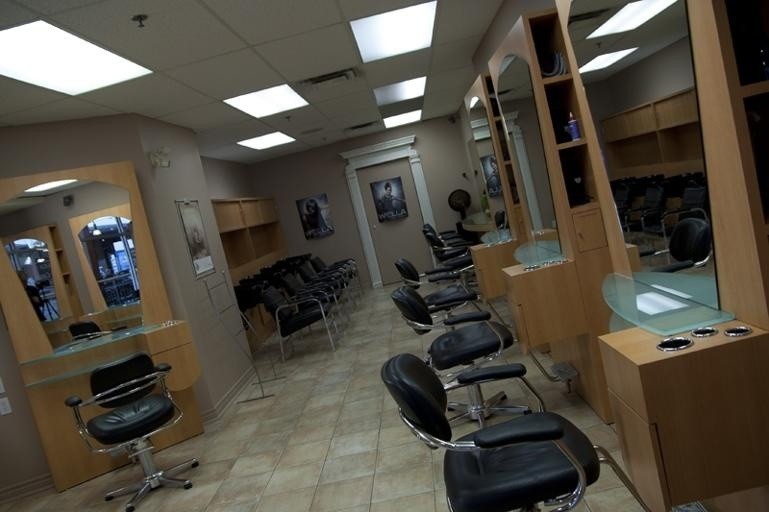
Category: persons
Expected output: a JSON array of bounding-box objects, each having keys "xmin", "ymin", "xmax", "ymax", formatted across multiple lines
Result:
[
  {"xmin": 481, "ymin": 157, "xmax": 502, "ymax": 190},
  {"xmin": 16, "ymin": 270, "xmax": 48, "ymax": 321},
  {"xmin": 373, "ymin": 182, "xmax": 406, "ymax": 213},
  {"xmin": 189, "ymin": 228, "xmax": 208, "ymax": 260},
  {"xmin": 299, "ymin": 199, "xmax": 330, "ymax": 230}
]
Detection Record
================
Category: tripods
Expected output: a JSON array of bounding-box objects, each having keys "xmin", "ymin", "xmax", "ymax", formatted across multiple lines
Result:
[{"xmin": 41, "ymin": 289, "xmax": 57, "ymax": 320}]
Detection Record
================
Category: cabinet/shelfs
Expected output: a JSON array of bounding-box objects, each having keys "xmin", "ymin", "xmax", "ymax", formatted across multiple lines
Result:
[
  {"xmin": 552, "ymin": 0, "xmax": 768, "ymax": 509},
  {"xmin": 209, "ymin": 194, "xmax": 296, "ymax": 352},
  {"xmin": 1, "ymin": 222, "xmax": 78, "ymax": 346},
  {"xmin": 457, "ymin": 73, "xmax": 530, "ymax": 307},
  {"xmin": 594, "ymin": 86, "xmax": 702, "ymax": 177},
  {"xmin": 69, "ymin": 200, "xmax": 138, "ymax": 317},
  {"xmin": 455, "ymin": 12, "xmax": 626, "ymax": 430},
  {"xmin": 1, "ymin": 160, "xmax": 207, "ymax": 494}
]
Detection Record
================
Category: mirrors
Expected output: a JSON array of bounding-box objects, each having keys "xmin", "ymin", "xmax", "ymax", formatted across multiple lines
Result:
[
  {"xmin": 496, "ymin": 51, "xmax": 563, "ymax": 259},
  {"xmin": 0, "ymin": 179, "xmax": 143, "ymax": 351},
  {"xmin": 469, "ymin": 95, "xmax": 512, "ymax": 240},
  {"xmin": 564, "ymin": 1, "xmax": 721, "ymax": 312}
]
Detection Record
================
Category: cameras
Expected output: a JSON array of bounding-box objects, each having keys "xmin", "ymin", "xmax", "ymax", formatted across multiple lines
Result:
[{"xmin": 35, "ymin": 279, "xmax": 51, "ymax": 289}]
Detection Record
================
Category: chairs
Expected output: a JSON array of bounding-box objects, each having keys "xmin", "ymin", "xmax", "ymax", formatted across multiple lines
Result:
[
  {"xmin": 603, "ymin": 170, "xmax": 714, "ymax": 282},
  {"xmin": 67, "ymin": 319, "xmax": 127, "ymax": 344},
  {"xmin": 232, "ymin": 250, "xmax": 368, "ymax": 365},
  {"xmin": 65, "ymin": 348, "xmax": 201, "ymax": 511}
]
